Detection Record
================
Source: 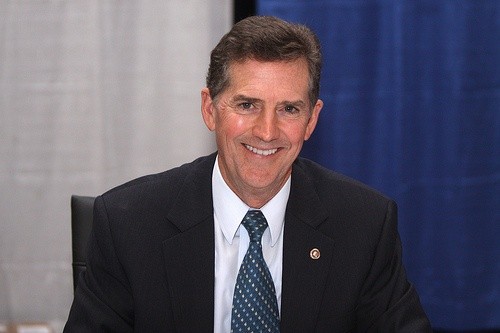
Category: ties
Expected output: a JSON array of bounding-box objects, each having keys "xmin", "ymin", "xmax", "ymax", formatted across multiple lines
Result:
[{"xmin": 231, "ymin": 210, "xmax": 282, "ymax": 333}]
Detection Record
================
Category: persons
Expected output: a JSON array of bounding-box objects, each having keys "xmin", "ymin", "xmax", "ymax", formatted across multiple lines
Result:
[{"xmin": 64, "ymin": 15, "xmax": 435, "ymax": 333}]
